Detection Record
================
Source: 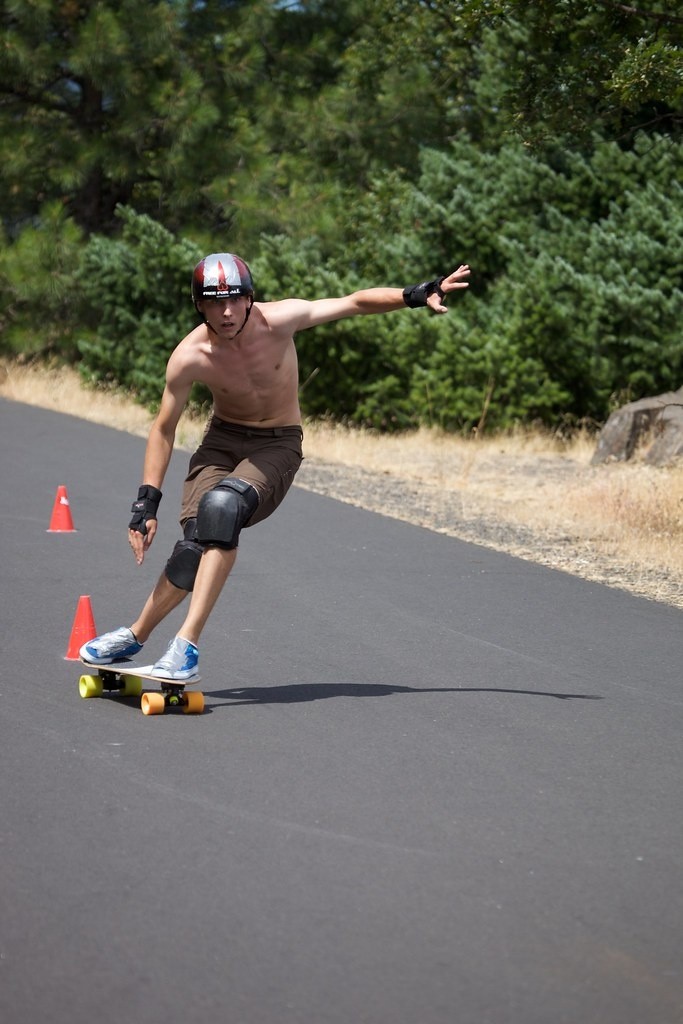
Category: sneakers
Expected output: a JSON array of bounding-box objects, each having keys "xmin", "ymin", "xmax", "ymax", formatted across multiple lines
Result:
[
  {"xmin": 151, "ymin": 636, "xmax": 199, "ymax": 679},
  {"xmin": 79, "ymin": 626, "xmax": 146, "ymax": 665}
]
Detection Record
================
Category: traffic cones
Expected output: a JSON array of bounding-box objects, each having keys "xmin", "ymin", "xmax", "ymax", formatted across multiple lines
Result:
[
  {"xmin": 45, "ymin": 485, "xmax": 77, "ymax": 533},
  {"xmin": 64, "ymin": 594, "xmax": 98, "ymax": 661}
]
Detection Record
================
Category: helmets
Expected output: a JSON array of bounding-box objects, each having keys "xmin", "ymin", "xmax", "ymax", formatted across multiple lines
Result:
[{"xmin": 191, "ymin": 253, "xmax": 255, "ymax": 303}]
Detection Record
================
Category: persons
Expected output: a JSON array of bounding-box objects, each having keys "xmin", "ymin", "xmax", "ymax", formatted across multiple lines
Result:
[{"xmin": 80, "ymin": 252, "xmax": 471, "ymax": 679}]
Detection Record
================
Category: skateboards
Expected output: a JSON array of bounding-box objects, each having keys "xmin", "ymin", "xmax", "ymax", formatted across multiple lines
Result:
[{"xmin": 78, "ymin": 654, "xmax": 204, "ymax": 715}]
